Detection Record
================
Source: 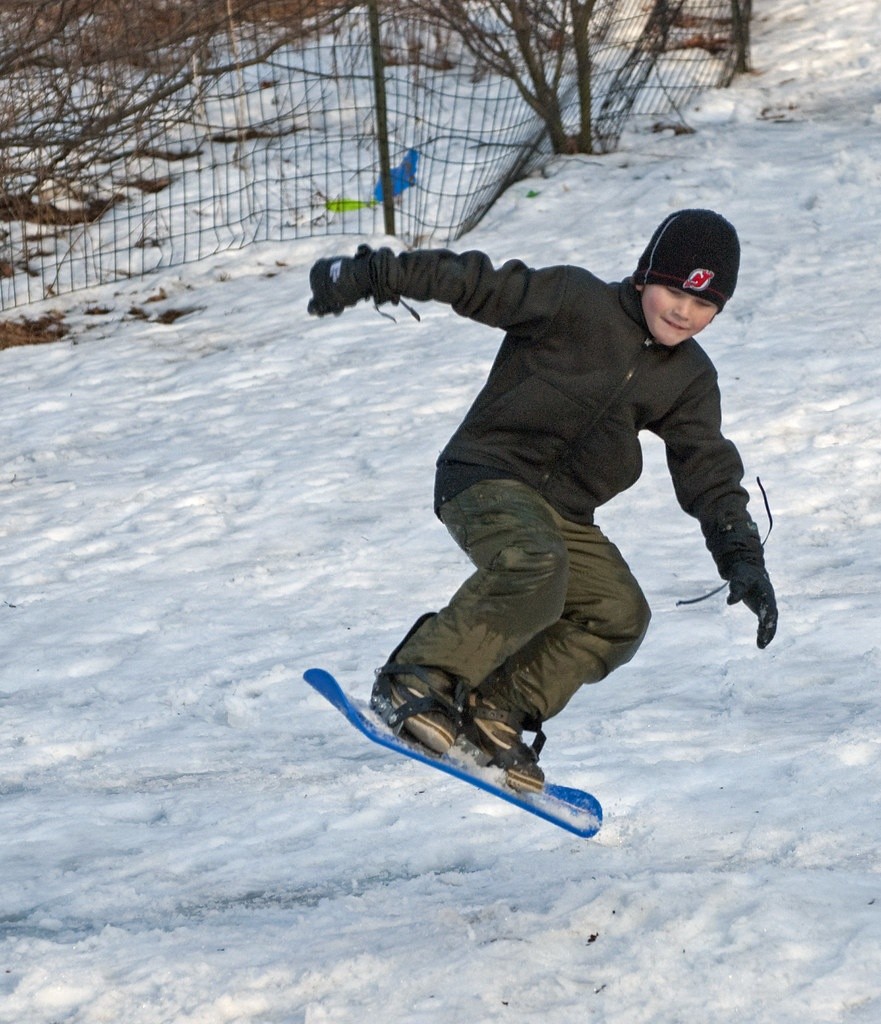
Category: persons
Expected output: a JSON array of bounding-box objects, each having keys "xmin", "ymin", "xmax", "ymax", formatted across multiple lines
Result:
[{"xmin": 307, "ymin": 205, "xmax": 778, "ymax": 792}]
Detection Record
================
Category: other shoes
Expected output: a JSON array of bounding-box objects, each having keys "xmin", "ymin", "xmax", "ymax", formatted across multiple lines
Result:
[
  {"xmin": 389, "ymin": 676, "xmax": 456, "ymax": 755},
  {"xmin": 462, "ymin": 691, "xmax": 546, "ymax": 793}
]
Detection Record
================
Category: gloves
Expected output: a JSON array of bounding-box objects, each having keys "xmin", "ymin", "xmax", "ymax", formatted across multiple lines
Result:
[
  {"xmin": 708, "ymin": 522, "xmax": 778, "ymax": 649},
  {"xmin": 307, "ymin": 251, "xmax": 377, "ymax": 315}
]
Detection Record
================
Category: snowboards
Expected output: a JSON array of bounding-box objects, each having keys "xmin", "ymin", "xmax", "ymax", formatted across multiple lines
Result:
[{"xmin": 298, "ymin": 666, "xmax": 607, "ymax": 841}]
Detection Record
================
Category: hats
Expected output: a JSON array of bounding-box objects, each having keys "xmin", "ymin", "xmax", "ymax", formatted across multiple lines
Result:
[{"xmin": 634, "ymin": 210, "xmax": 740, "ymax": 315}]
